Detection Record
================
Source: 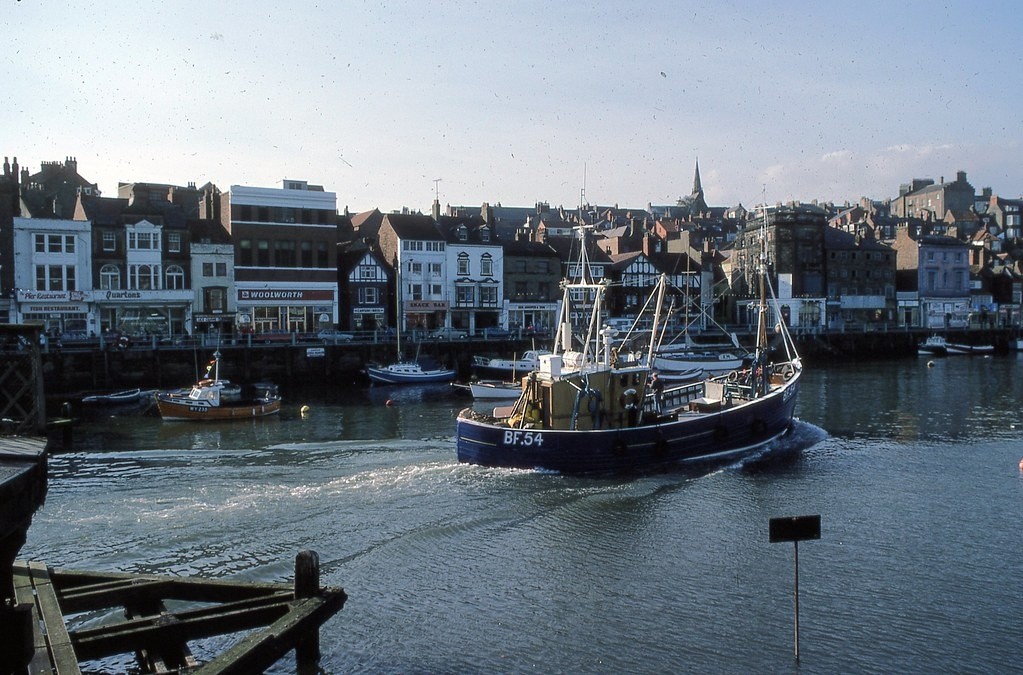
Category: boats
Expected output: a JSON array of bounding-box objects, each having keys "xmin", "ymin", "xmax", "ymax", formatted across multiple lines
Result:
[
  {"xmin": 917, "ymin": 333, "xmax": 1023, "ymax": 356},
  {"xmin": 250, "ymin": 379, "xmax": 280, "ymax": 400},
  {"xmin": 455, "ymin": 163, "xmax": 803, "ymax": 472},
  {"xmin": 47, "ymin": 415, "xmax": 79, "ymax": 434},
  {"xmin": 582, "ymin": 318, "xmax": 654, "ymax": 351},
  {"xmin": 153, "ymin": 328, "xmax": 283, "ymax": 422},
  {"xmin": 82, "ymin": 388, "xmax": 142, "ymax": 407},
  {"xmin": 468, "ymin": 348, "xmax": 563, "ymax": 381}
]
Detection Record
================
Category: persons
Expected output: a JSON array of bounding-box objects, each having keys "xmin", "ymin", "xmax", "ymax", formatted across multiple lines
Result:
[
  {"xmin": 588, "ymin": 386, "xmax": 604, "ymax": 430},
  {"xmin": 647, "ymin": 372, "xmax": 665, "ymax": 415}
]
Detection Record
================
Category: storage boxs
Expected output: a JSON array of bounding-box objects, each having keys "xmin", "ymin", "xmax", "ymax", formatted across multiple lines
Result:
[{"xmin": 688, "ymin": 382, "xmax": 724, "ymax": 413}]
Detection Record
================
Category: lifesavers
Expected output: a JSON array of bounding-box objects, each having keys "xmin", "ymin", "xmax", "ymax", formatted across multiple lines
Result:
[
  {"xmin": 620, "ymin": 388, "xmax": 639, "ymax": 410},
  {"xmin": 728, "ymin": 370, "xmax": 738, "ymax": 382},
  {"xmin": 783, "ymin": 370, "xmax": 795, "ymax": 381}
]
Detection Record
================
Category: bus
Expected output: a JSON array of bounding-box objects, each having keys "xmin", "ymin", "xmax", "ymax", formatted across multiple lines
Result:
[{"xmin": 966, "ymin": 312, "xmax": 1008, "ymax": 331}]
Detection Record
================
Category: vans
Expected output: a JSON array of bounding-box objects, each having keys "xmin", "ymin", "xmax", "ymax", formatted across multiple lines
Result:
[{"xmin": 657, "ymin": 313, "xmax": 704, "ymax": 334}]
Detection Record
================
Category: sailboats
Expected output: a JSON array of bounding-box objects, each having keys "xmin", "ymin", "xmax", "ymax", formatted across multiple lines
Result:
[
  {"xmin": 639, "ymin": 244, "xmax": 747, "ymax": 371},
  {"xmin": 366, "ymin": 270, "xmax": 455, "ymax": 385}
]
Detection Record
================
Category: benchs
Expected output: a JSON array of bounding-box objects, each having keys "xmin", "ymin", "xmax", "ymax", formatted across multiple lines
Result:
[{"xmin": 492, "ymin": 406, "xmax": 512, "ymax": 423}]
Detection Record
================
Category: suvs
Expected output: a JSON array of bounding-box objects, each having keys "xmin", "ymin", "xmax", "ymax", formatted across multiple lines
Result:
[
  {"xmin": 480, "ymin": 326, "xmax": 512, "ymax": 338},
  {"xmin": 526, "ymin": 325, "xmax": 557, "ymax": 338},
  {"xmin": 317, "ymin": 328, "xmax": 355, "ymax": 344}
]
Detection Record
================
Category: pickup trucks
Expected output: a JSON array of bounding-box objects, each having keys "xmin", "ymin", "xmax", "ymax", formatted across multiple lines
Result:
[{"xmin": 428, "ymin": 326, "xmax": 468, "ymax": 340}]
